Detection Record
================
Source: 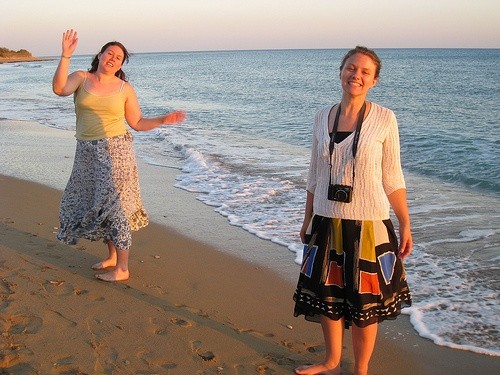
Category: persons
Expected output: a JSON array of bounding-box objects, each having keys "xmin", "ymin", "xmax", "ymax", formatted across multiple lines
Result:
[
  {"xmin": 292, "ymin": 45, "xmax": 415, "ymax": 375},
  {"xmin": 50, "ymin": 27, "xmax": 187, "ymax": 284}
]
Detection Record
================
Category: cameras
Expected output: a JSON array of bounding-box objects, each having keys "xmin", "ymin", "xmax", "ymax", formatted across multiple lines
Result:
[{"xmin": 328, "ymin": 183, "xmax": 353, "ymax": 203}]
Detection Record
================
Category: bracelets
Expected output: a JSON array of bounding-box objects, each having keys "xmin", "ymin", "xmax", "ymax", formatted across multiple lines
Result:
[{"xmin": 62, "ymin": 55, "xmax": 71, "ymax": 59}]
